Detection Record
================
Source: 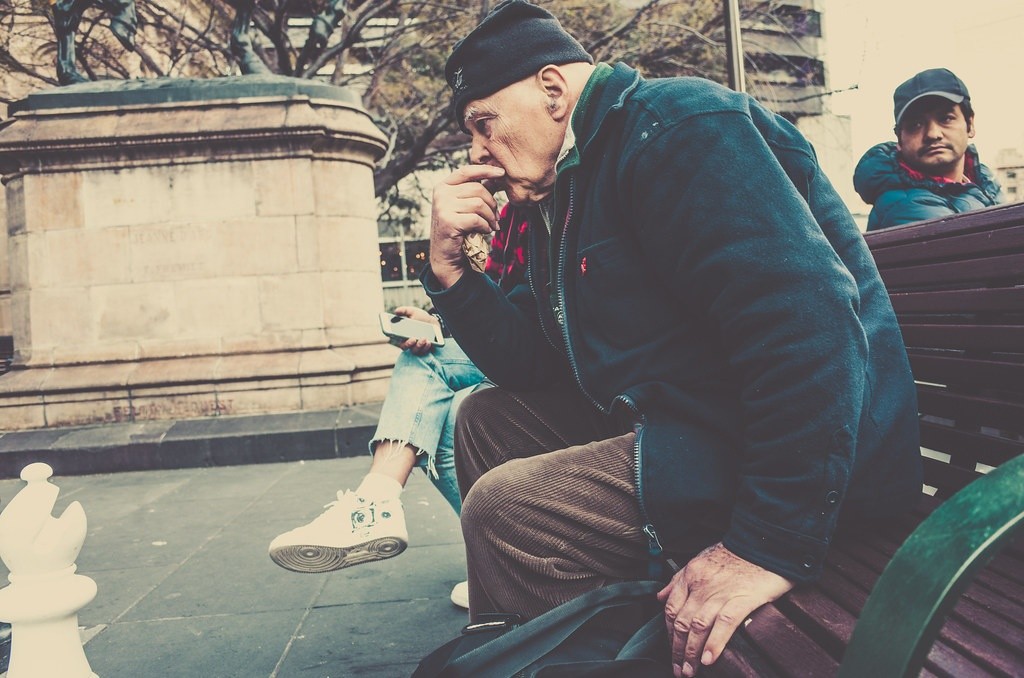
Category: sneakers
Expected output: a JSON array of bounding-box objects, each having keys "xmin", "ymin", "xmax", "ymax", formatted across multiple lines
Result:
[
  {"xmin": 450, "ymin": 580, "xmax": 469, "ymax": 608},
  {"xmin": 267, "ymin": 487, "xmax": 408, "ymax": 573}
]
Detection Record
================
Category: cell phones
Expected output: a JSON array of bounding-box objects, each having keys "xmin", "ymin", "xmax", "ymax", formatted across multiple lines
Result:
[{"xmin": 380, "ymin": 312, "xmax": 444, "ymax": 348}]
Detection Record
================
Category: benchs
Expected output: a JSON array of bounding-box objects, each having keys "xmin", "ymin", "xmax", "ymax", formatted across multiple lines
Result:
[{"xmin": 702, "ymin": 202, "xmax": 1024, "ymax": 678}]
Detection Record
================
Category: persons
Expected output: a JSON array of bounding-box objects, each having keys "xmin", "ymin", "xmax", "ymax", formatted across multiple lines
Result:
[
  {"xmin": 852, "ymin": 67, "xmax": 1005, "ymax": 231},
  {"xmin": 267, "ymin": 0, "xmax": 922, "ymax": 678}
]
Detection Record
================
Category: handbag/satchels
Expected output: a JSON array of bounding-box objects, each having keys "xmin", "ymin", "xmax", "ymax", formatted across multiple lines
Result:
[{"xmin": 410, "ymin": 581, "xmax": 748, "ymax": 678}]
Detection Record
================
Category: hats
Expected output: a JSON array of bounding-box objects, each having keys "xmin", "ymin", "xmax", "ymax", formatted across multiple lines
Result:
[
  {"xmin": 893, "ymin": 68, "xmax": 970, "ymax": 123},
  {"xmin": 444, "ymin": 0, "xmax": 594, "ymax": 135}
]
